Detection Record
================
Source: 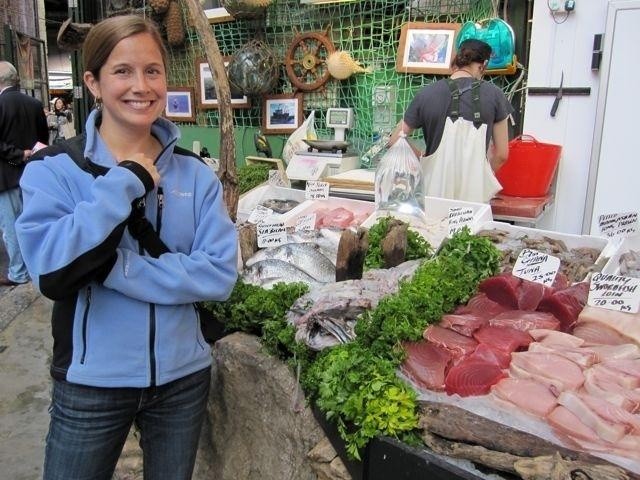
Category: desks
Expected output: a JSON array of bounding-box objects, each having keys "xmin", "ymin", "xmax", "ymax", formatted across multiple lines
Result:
[{"xmin": 313, "ymin": 405, "xmax": 508, "ymax": 480}]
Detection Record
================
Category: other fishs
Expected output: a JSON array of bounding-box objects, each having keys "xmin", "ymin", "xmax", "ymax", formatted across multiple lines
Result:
[{"xmin": 244, "ymin": 197, "xmax": 365, "ymax": 295}]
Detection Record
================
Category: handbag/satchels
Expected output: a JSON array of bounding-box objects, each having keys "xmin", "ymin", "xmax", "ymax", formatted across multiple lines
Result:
[
  {"xmin": 60, "ymin": 120, "xmax": 76, "ymax": 141},
  {"xmin": 193, "ymin": 301, "xmax": 226, "ymax": 342}
]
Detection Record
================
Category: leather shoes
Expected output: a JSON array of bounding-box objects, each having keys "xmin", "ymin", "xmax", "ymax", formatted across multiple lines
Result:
[{"xmin": 0, "ymin": 276, "xmax": 29, "ymax": 286}]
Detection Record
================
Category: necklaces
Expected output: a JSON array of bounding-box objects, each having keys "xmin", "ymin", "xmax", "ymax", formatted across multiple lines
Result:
[{"xmin": 452, "ymin": 69, "xmax": 474, "ymax": 77}]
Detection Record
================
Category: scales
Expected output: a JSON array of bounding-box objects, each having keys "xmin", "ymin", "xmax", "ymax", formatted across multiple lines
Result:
[{"xmin": 286, "ymin": 108, "xmax": 360, "ymax": 181}]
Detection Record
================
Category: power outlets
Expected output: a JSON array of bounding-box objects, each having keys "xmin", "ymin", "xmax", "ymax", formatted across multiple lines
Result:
[{"xmin": 549, "ymin": 0, "xmax": 574, "ymax": 14}]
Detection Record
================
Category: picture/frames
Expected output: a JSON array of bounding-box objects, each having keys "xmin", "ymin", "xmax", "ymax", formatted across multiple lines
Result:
[
  {"xmin": 262, "ymin": 92, "xmax": 304, "ymax": 135},
  {"xmin": 162, "ymin": 87, "xmax": 195, "ymax": 122},
  {"xmin": 187, "ymin": 0, "xmax": 236, "ymax": 26},
  {"xmin": 196, "ymin": 56, "xmax": 251, "ymax": 109},
  {"xmin": 395, "ymin": 21, "xmax": 461, "ymax": 75}
]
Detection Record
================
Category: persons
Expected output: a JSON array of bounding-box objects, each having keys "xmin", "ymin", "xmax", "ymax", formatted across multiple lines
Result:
[
  {"xmin": 200, "ymin": 146, "xmax": 211, "ymax": 158},
  {"xmin": 0, "ymin": 58, "xmax": 51, "ymax": 285},
  {"xmin": 43, "ymin": 95, "xmax": 76, "ymax": 148},
  {"xmin": 388, "ymin": 36, "xmax": 516, "ymax": 212},
  {"xmin": 13, "ymin": 12, "xmax": 240, "ymax": 480},
  {"xmin": 171, "ymin": 98, "xmax": 180, "ymax": 112}
]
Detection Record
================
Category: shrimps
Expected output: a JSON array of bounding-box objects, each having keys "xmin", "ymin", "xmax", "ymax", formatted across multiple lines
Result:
[{"xmin": 477, "ymin": 227, "xmax": 611, "ymax": 290}]
